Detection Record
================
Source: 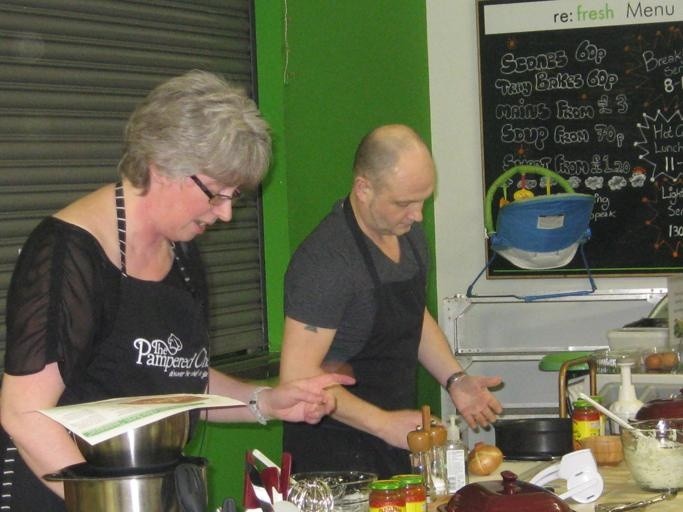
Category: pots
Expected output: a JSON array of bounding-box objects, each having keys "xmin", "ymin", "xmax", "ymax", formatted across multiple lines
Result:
[
  {"xmin": 491, "ymin": 418, "xmax": 574, "ymax": 457},
  {"xmin": 43, "ymin": 457, "xmax": 209, "ymax": 512}
]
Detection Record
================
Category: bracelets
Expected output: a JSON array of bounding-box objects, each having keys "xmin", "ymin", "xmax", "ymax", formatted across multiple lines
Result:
[
  {"xmin": 443, "ymin": 372, "xmax": 469, "ymax": 391},
  {"xmin": 249, "ymin": 385, "xmax": 274, "ymax": 428}
]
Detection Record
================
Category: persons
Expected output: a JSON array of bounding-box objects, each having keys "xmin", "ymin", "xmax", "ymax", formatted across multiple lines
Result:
[
  {"xmin": 279, "ymin": 122, "xmax": 504, "ymax": 480},
  {"xmin": 2, "ymin": 72, "xmax": 356, "ymax": 512}
]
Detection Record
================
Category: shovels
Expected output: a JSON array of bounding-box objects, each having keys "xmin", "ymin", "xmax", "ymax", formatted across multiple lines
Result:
[
  {"xmin": 571, "ymin": 391, "xmax": 673, "ymax": 457},
  {"xmin": 168, "ymin": 458, "xmax": 217, "ymax": 510}
]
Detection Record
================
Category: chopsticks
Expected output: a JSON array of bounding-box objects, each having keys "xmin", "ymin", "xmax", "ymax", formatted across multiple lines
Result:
[
  {"xmin": 252, "ymin": 448, "xmax": 311, "ymax": 501},
  {"xmin": 579, "ymin": 392, "xmax": 647, "ymax": 438}
]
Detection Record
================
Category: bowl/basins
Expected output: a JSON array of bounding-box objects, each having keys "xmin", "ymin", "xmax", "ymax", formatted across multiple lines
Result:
[
  {"xmin": 620, "ymin": 417, "xmax": 683, "ymax": 492},
  {"xmin": 291, "ymin": 471, "xmax": 378, "ymax": 511},
  {"xmin": 72, "ymin": 410, "xmax": 190, "ymax": 477},
  {"xmin": 641, "ymin": 345, "xmax": 682, "ymax": 371}
]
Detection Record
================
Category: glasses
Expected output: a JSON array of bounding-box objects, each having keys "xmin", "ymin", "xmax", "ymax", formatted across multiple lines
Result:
[{"xmin": 189, "ymin": 174, "xmax": 243, "ymax": 206}]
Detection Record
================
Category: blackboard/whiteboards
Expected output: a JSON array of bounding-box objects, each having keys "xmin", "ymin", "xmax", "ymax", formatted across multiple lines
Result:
[{"xmin": 475, "ymin": 1, "xmax": 683, "ymax": 279}]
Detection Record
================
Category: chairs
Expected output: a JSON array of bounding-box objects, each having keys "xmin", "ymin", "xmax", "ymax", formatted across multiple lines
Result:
[{"xmin": 465, "ymin": 168, "xmax": 597, "ymax": 302}]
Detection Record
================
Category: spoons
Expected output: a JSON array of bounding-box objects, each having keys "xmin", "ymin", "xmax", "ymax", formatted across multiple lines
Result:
[{"xmin": 595, "ymin": 491, "xmax": 678, "ymax": 512}]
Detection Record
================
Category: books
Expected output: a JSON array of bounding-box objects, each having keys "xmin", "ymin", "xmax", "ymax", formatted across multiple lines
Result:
[{"xmin": 20, "ymin": 393, "xmax": 252, "ymax": 446}]
